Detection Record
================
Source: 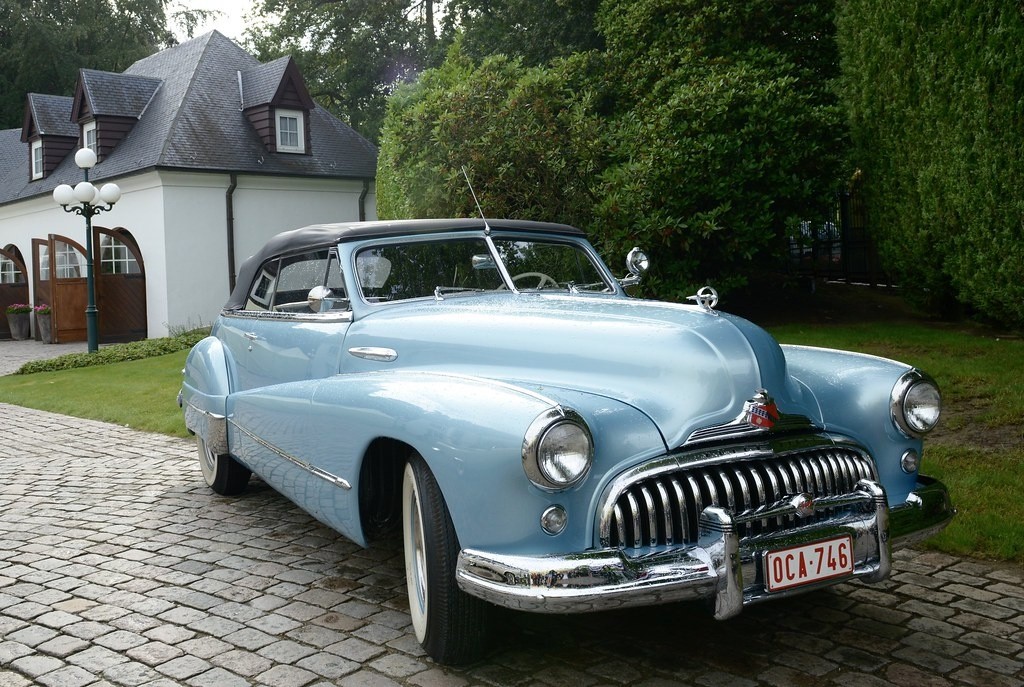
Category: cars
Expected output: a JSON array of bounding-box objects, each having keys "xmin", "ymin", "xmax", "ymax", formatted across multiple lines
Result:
[{"xmin": 175, "ymin": 216, "xmax": 961, "ymax": 670}]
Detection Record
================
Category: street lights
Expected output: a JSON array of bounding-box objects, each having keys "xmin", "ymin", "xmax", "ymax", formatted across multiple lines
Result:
[{"xmin": 52, "ymin": 147, "xmax": 121, "ymax": 355}]
